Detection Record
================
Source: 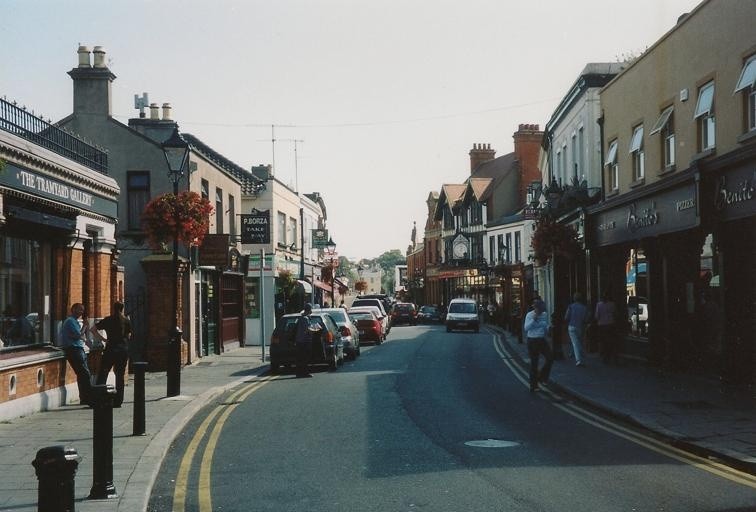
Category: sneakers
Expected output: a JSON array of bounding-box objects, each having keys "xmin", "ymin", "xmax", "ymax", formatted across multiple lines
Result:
[
  {"xmin": 533, "ymin": 379, "xmax": 549, "ymax": 392},
  {"xmin": 575, "ymin": 361, "xmax": 584, "ymax": 367}
]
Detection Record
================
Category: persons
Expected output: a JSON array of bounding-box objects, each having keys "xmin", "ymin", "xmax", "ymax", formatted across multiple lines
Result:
[
  {"xmin": 320, "ymin": 301, "xmax": 330, "ymax": 309},
  {"xmin": 529, "ymin": 290, "xmax": 543, "ymax": 303},
  {"xmin": 88, "ymin": 300, "xmax": 135, "ymax": 407},
  {"xmin": 524, "ymin": 299, "xmax": 557, "ymax": 393},
  {"xmin": 564, "ymin": 292, "xmax": 591, "ymax": 366},
  {"xmin": 4, "ymin": 304, "xmax": 35, "ymax": 346},
  {"xmin": 339, "ymin": 300, "xmax": 347, "ymax": 311},
  {"xmin": 294, "ymin": 302, "xmax": 322, "ymax": 378},
  {"xmin": 588, "ymin": 290, "xmax": 618, "ymax": 363},
  {"xmin": 61, "ymin": 303, "xmax": 92, "ymax": 406},
  {"xmin": 487, "ymin": 300, "xmax": 497, "ymax": 331}
]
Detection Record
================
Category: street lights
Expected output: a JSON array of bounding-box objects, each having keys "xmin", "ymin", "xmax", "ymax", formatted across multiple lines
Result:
[
  {"xmin": 326, "ymin": 238, "xmax": 337, "ymax": 306},
  {"xmin": 357, "ymin": 266, "xmax": 364, "ymax": 295},
  {"xmin": 159, "ymin": 122, "xmax": 191, "ymax": 400}
]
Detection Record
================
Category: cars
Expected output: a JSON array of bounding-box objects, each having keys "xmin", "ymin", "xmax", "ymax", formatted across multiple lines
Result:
[
  {"xmin": 631, "ymin": 303, "xmax": 651, "ymax": 334},
  {"xmin": 267, "ymin": 292, "xmax": 441, "ymax": 372}
]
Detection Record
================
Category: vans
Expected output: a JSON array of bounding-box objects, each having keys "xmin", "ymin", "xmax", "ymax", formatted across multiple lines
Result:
[{"xmin": 446, "ymin": 297, "xmax": 481, "ymax": 334}]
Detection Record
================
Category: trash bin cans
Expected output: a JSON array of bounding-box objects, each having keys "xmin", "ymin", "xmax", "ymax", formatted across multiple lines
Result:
[{"xmin": 31, "ymin": 445, "xmax": 82, "ymax": 512}]
